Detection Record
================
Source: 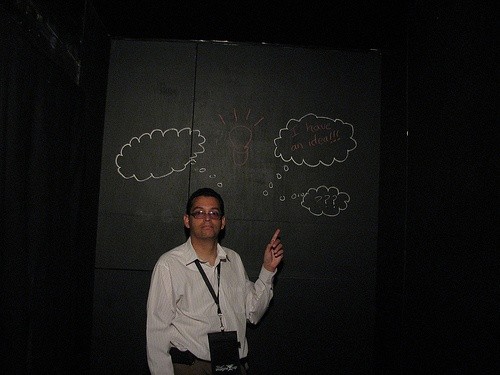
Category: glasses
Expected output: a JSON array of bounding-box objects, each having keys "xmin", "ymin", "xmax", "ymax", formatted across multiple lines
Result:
[{"xmin": 188, "ymin": 209, "xmax": 223, "ymax": 219}]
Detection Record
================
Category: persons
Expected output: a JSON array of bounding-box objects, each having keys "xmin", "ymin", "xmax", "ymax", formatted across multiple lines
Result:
[{"xmin": 145, "ymin": 187, "xmax": 284, "ymax": 375}]
